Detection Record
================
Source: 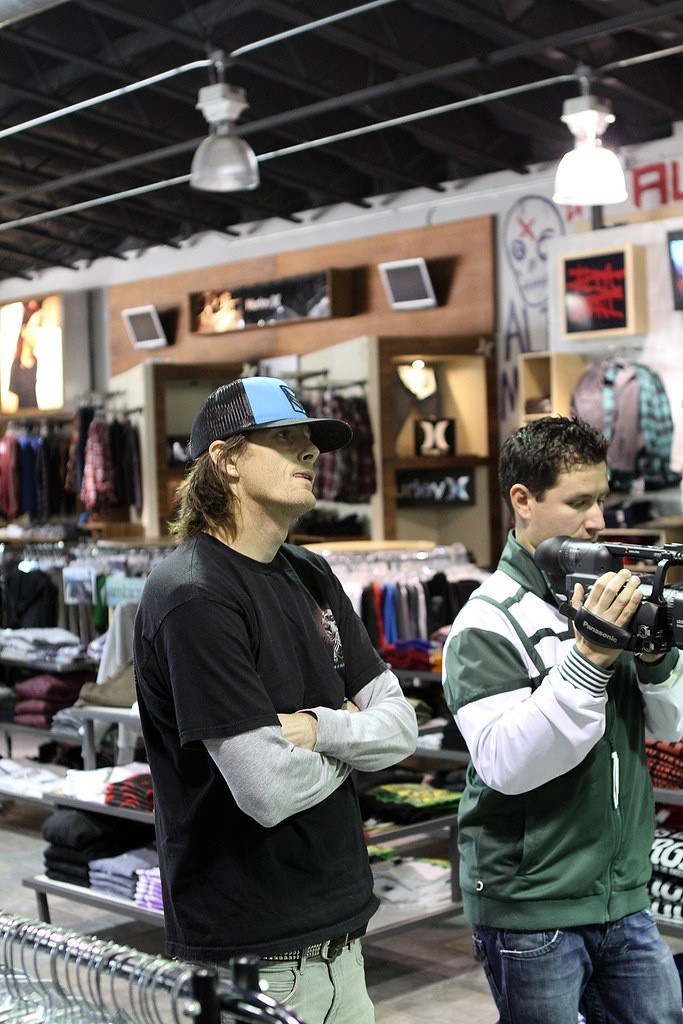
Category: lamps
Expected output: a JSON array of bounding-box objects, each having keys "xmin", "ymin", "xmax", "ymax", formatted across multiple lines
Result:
[
  {"xmin": 189, "ymin": 49, "xmax": 259, "ymax": 192},
  {"xmin": 553, "ymin": 66, "xmax": 628, "ymax": 206}
]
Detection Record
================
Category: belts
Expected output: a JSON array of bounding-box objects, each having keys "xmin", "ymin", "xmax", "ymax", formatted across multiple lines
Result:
[{"xmin": 259, "ymin": 920, "xmax": 369, "ymax": 963}]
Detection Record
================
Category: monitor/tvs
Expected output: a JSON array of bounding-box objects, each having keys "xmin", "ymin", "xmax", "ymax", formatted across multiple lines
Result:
[
  {"xmin": 378, "ymin": 256, "xmax": 437, "ymax": 311},
  {"xmin": 121, "ymin": 304, "xmax": 168, "ymax": 351}
]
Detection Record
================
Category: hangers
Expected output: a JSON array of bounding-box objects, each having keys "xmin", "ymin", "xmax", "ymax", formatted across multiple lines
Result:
[{"xmin": 0, "ymin": 911, "xmax": 203, "ymax": 1024}]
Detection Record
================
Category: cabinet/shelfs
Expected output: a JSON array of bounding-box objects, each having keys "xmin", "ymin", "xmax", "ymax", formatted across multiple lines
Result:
[
  {"xmin": 519, "ymin": 351, "xmax": 683, "ymax": 585},
  {"xmin": 0, "ymin": 706, "xmax": 464, "ymax": 946},
  {"xmin": 652, "ymin": 786, "xmax": 683, "ymax": 937}
]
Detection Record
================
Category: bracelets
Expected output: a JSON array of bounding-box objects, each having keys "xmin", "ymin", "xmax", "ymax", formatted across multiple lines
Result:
[{"xmin": 341, "ymin": 694, "xmax": 365, "ymax": 710}]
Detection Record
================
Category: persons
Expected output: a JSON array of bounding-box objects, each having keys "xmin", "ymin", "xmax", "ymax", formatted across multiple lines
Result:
[
  {"xmin": 441, "ymin": 416, "xmax": 683, "ymax": 1024},
  {"xmin": 133, "ymin": 375, "xmax": 418, "ymax": 1024}
]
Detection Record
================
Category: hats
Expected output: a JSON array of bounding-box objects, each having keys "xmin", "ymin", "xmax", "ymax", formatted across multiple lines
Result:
[{"xmin": 189, "ymin": 376, "xmax": 353, "ymax": 460}]
